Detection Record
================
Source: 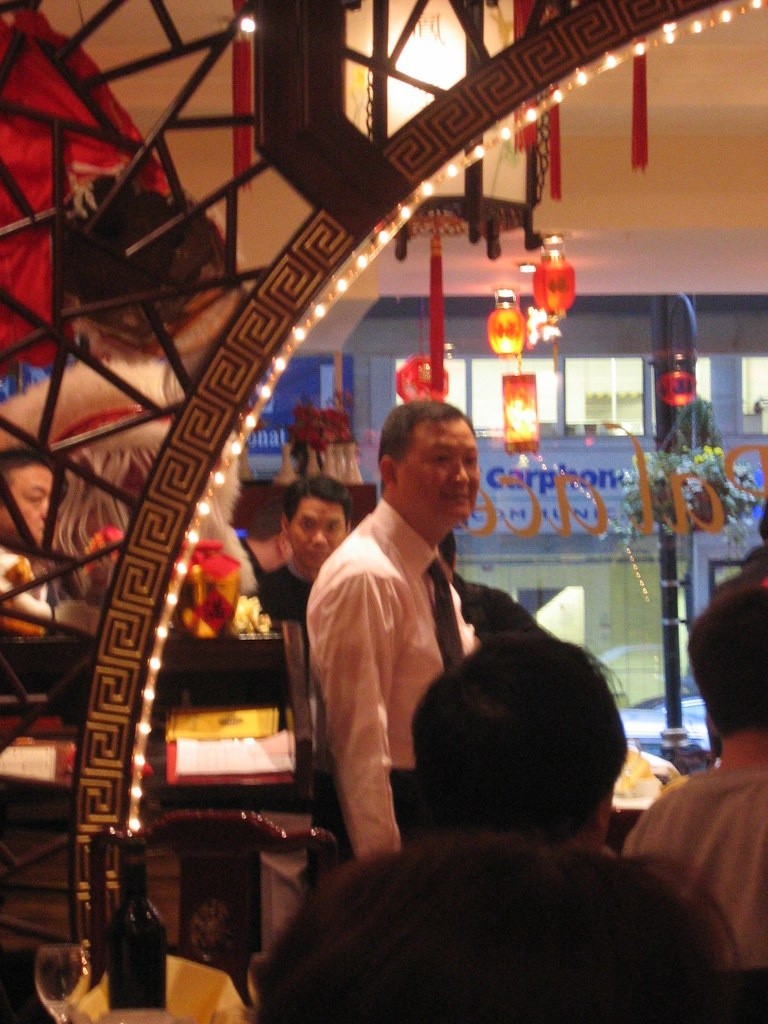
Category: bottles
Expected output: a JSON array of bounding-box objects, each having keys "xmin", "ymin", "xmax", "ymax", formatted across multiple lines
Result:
[{"xmin": 659, "ymin": 728, "xmax": 688, "ymax": 761}]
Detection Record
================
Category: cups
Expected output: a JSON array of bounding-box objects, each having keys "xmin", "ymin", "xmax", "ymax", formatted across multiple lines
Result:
[{"xmin": 34, "ymin": 944, "xmax": 92, "ymax": 1024}]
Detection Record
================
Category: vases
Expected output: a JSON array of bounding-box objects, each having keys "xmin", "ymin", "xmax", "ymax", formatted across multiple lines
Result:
[
  {"xmin": 650, "ymin": 482, "xmax": 726, "ymax": 533},
  {"xmin": 273, "ymin": 441, "xmax": 364, "ymax": 486},
  {"xmin": 238, "ymin": 447, "xmax": 254, "ymax": 481}
]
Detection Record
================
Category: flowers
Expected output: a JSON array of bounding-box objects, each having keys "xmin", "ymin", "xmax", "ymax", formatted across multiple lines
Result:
[
  {"xmin": 240, "ymin": 405, "xmax": 267, "ymax": 432},
  {"xmin": 285, "ymin": 386, "xmax": 353, "ymax": 452},
  {"xmin": 623, "ymin": 445, "xmax": 761, "ymax": 560}
]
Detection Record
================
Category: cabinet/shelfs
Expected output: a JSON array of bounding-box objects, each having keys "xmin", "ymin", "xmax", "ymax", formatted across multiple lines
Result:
[{"xmin": 0, "ymin": 618, "xmax": 312, "ymax": 950}]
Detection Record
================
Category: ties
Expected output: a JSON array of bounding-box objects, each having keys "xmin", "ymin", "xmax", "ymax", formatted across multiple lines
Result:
[{"xmin": 428, "ymin": 560, "xmax": 464, "ymax": 668}]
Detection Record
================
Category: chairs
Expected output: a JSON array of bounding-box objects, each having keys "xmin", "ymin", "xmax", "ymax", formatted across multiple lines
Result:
[{"xmin": 86, "ymin": 811, "xmax": 339, "ymax": 1008}]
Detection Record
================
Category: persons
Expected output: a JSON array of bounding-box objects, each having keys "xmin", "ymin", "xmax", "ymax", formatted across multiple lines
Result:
[
  {"xmin": 249, "ymin": 825, "xmax": 732, "ymax": 1023},
  {"xmin": 630, "ymin": 489, "xmax": 768, "ymax": 1024},
  {"xmin": 1, "ymin": 445, "xmax": 59, "ymax": 638},
  {"xmin": 241, "ymin": 483, "xmax": 298, "ymax": 592},
  {"xmin": 257, "ymin": 473, "xmax": 356, "ymax": 744},
  {"xmin": 403, "ymin": 627, "xmax": 628, "ymax": 859},
  {"xmin": 307, "ymin": 399, "xmax": 486, "ymax": 879},
  {"xmin": 436, "ymin": 522, "xmax": 546, "ymax": 633}
]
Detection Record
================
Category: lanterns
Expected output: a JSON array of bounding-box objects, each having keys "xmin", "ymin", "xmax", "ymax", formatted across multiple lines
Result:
[
  {"xmin": 656, "ymin": 369, "xmax": 697, "ymax": 408},
  {"xmin": 486, "ymin": 291, "xmax": 527, "ymax": 359},
  {"xmin": 393, "ymin": 355, "xmax": 449, "ymax": 406},
  {"xmin": 531, "ymin": 254, "xmax": 577, "ymax": 323},
  {"xmin": 504, "ymin": 373, "xmax": 539, "ymax": 457},
  {"xmin": 345, "ymin": 0, "xmax": 537, "ymax": 263}
]
